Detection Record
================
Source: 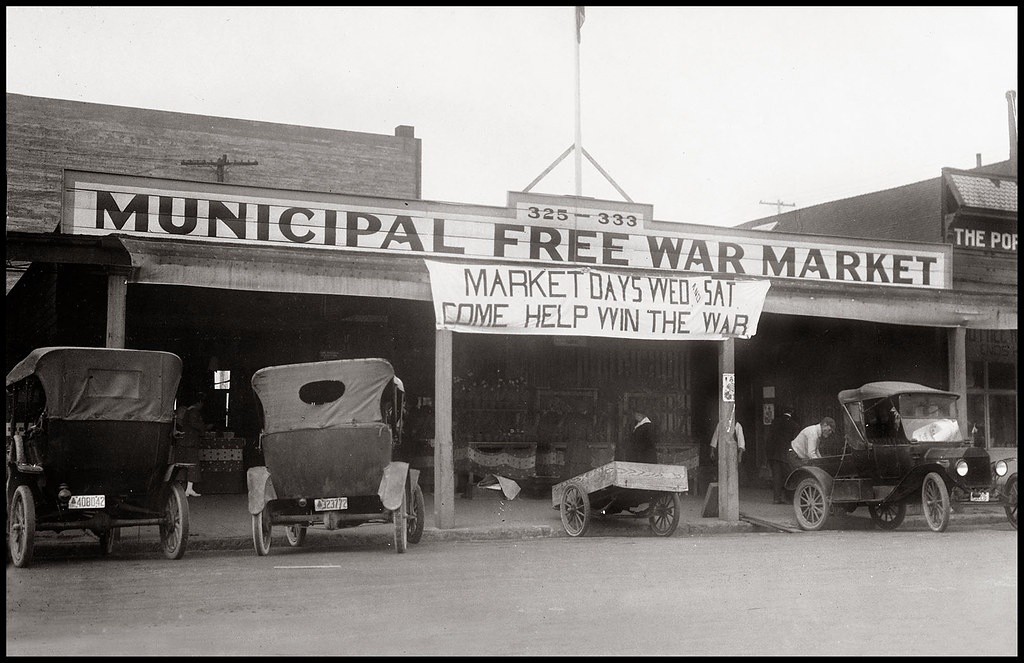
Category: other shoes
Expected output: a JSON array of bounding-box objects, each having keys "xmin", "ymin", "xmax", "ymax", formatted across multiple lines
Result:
[
  {"xmin": 185, "ymin": 490, "xmax": 202, "ymax": 497},
  {"xmin": 774, "ymin": 498, "xmax": 782, "ymax": 504}
]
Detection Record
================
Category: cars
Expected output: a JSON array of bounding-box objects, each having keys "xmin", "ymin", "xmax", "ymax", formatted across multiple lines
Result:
[
  {"xmin": 782, "ymin": 380, "xmax": 1019, "ymax": 535},
  {"xmin": 1, "ymin": 347, "xmax": 199, "ymax": 569},
  {"xmin": 245, "ymin": 355, "xmax": 424, "ymax": 557}
]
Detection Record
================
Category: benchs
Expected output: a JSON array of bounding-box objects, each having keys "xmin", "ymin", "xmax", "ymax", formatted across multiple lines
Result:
[{"xmin": 852, "ymin": 420, "xmax": 909, "ymax": 445}]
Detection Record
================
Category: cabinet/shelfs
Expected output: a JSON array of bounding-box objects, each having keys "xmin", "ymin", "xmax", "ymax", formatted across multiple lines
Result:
[{"xmin": 534, "ymin": 385, "xmax": 598, "ymax": 447}]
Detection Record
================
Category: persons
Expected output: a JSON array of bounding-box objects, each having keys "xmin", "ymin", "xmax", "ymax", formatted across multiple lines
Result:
[
  {"xmin": 627, "ymin": 405, "xmax": 659, "ymax": 466},
  {"xmin": 178, "ymin": 392, "xmax": 213, "ymax": 497},
  {"xmin": 710, "ymin": 420, "xmax": 746, "ymax": 464},
  {"xmin": 765, "ymin": 407, "xmax": 836, "ymax": 504},
  {"xmin": 557, "ymin": 398, "xmax": 599, "ymax": 478}
]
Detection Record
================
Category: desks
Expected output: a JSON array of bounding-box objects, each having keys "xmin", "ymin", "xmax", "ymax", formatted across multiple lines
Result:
[{"xmin": 198, "ymin": 438, "xmax": 247, "ymax": 493}]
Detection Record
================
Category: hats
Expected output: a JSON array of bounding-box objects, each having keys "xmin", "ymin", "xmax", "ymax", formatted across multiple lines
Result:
[{"xmin": 825, "ymin": 417, "xmax": 836, "ymax": 434}]
